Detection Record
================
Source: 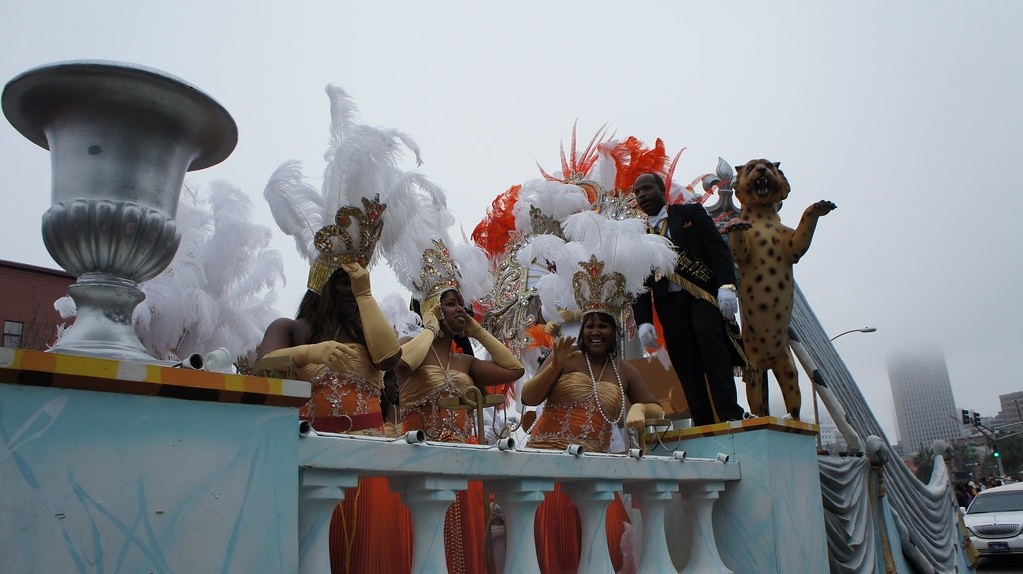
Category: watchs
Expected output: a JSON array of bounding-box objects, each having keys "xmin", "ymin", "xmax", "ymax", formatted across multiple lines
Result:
[{"xmin": 720, "ymin": 284, "xmax": 736, "ymax": 290}]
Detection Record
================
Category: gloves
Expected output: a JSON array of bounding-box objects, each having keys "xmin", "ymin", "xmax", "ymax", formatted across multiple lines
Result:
[
  {"xmin": 464, "ymin": 314, "xmax": 525, "ymax": 380},
  {"xmin": 399, "ymin": 304, "xmax": 440, "ymax": 374},
  {"xmin": 342, "ymin": 261, "xmax": 401, "ymax": 364},
  {"xmin": 638, "ymin": 322, "xmax": 658, "ymax": 349},
  {"xmin": 521, "ymin": 336, "xmax": 582, "ymax": 404},
  {"xmin": 717, "ymin": 285, "xmax": 738, "ymax": 319},
  {"xmin": 626, "ymin": 403, "xmax": 663, "ymax": 435},
  {"xmin": 262, "ymin": 340, "xmax": 358, "ymax": 373}
]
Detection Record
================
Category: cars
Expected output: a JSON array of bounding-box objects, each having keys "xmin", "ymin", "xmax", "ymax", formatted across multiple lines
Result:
[{"xmin": 959, "ymin": 482, "xmax": 1023, "ymax": 556}]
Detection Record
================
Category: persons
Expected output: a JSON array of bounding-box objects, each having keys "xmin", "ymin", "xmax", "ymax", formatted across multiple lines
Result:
[
  {"xmin": 395, "ymin": 289, "xmax": 524, "ymax": 574},
  {"xmin": 258, "ymin": 254, "xmax": 413, "ymax": 573},
  {"xmin": 381, "ymin": 370, "xmax": 402, "ymax": 438},
  {"xmin": 629, "ymin": 172, "xmax": 744, "ymax": 424},
  {"xmin": 953, "ymin": 475, "xmax": 1018, "ymax": 508},
  {"xmin": 522, "ymin": 303, "xmax": 663, "ymax": 574}
]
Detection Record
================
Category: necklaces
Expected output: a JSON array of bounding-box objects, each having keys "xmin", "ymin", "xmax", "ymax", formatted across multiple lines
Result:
[{"xmin": 585, "ymin": 351, "xmax": 624, "ymax": 424}]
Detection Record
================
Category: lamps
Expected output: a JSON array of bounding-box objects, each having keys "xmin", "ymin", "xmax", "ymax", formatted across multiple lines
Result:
[
  {"xmin": 172, "ymin": 352, "xmax": 205, "ymax": 371},
  {"xmin": 717, "ymin": 452, "xmax": 729, "ymax": 463},
  {"xmin": 204, "ymin": 347, "xmax": 233, "ymax": 371},
  {"xmin": 299, "ymin": 420, "xmax": 311, "ymax": 437},
  {"xmin": 405, "ymin": 429, "xmax": 426, "ymax": 444},
  {"xmin": 567, "ymin": 444, "xmax": 583, "ymax": 455},
  {"xmin": 628, "ymin": 448, "xmax": 643, "ymax": 458},
  {"xmin": 497, "ymin": 438, "xmax": 515, "ymax": 451},
  {"xmin": 673, "ymin": 451, "xmax": 687, "ymax": 460},
  {"xmin": 743, "ymin": 412, "xmax": 755, "ymax": 420}
]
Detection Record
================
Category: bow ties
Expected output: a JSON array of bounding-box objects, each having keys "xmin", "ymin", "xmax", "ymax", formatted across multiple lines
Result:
[{"xmin": 649, "ymin": 207, "xmax": 668, "ymax": 227}]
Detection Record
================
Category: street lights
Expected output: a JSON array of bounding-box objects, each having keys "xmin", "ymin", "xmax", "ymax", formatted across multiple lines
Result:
[
  {"xmin": 994, "ymin": 451, "xmax": 999, "ymax": 458},
  {"xmin": 812, "ymin": 325, "xmax": 876, "ymax": 457}
]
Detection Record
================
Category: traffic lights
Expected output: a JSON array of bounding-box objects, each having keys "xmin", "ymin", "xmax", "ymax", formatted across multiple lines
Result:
[
  {"xmin": 973, "ymin": 413, "xmax": 981, "ymax": 426},
  {"xmin": 962, "ymin": 410, "xmax": 969, "ymax": 425}
]
